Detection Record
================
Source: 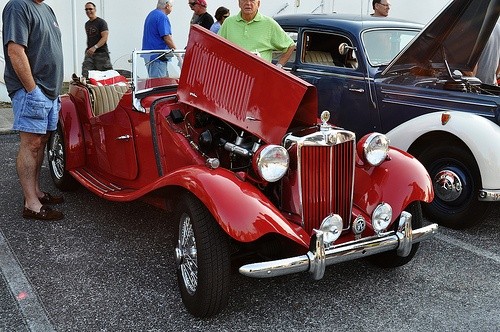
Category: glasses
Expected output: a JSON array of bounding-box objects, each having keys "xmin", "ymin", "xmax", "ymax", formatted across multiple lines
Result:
[
  {"xmin": 85, "ymin": 8, "xmax": 92, "ymax": 10},
  {"xmin": 223, "ymin": 15, "xmax": 228, "ymax": 18},
  {"xmin": 192, "ymin": 3, "xmax": 196, "ymax": 5},
  {"xmin": 380, "ymin": 3, "xmax": 391, "ymax": 6}
]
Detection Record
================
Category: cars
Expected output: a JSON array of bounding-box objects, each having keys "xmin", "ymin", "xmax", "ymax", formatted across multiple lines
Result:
[{"xmin": 268, "ymin": 0, "xmax": 500, "ymax": 226}]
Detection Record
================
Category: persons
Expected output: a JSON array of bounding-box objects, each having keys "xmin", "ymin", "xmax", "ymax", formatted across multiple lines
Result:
[
  {"xmin": 216, "ymin": 0, "xmax": 296, "ymax": 71},
  {"xmin": 82, "ymin": 2, "xmax": 113, "ymax": 79},
  {"xmin": 139, "ymin": 0, "xmax": 183, "ymax": 79},
  {"xmin": 184, "ymin": 0, "xmax": 230, "ymax": 50},
  {"xmin": 463, "ymin": 23, "xmax": 500, "ymax": 87},
  {"xmin": 0, "ymin": 0, "xmax": 66, "ymax": 221},
  {"xmin": 370, "ymin": 0, "xmax": 390, "ymax": 17}
]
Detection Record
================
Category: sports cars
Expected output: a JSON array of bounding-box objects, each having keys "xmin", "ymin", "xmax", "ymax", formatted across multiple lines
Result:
[{"xmin": 46, "ymin": 22, "xmax": 441, "ymax": 320}]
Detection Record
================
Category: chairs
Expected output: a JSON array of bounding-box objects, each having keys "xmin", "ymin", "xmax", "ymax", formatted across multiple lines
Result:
[
  {"xmin": 305, "ymin": 50, "xmax": 336, "ymax": 67},
  {"xmin": 89, "ymin": 85, "xmax": 128, "ymax": 116}
]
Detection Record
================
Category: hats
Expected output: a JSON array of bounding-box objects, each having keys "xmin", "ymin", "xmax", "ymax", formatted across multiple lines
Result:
[{"xmin": 195, "ymin": 0, "xmax": 207, "ymax": 7}]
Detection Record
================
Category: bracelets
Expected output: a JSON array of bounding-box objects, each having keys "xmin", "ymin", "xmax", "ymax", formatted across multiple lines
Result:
[
  {"xmin": 94, "ymin": 45, "xmax": 98, "ymax": 49},
  {"xmin": 276, "ymin": 63, "xmax": 283, "ymax": 69}
]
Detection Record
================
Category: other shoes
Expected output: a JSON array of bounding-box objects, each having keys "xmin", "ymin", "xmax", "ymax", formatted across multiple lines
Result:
[
  {"xmin": 40, "ymin": 192, "xmax": 63, "ymax": 204},
  {"xmin": 23, "ymin": 206, "xmax": 63, "ymax": 220}
]
